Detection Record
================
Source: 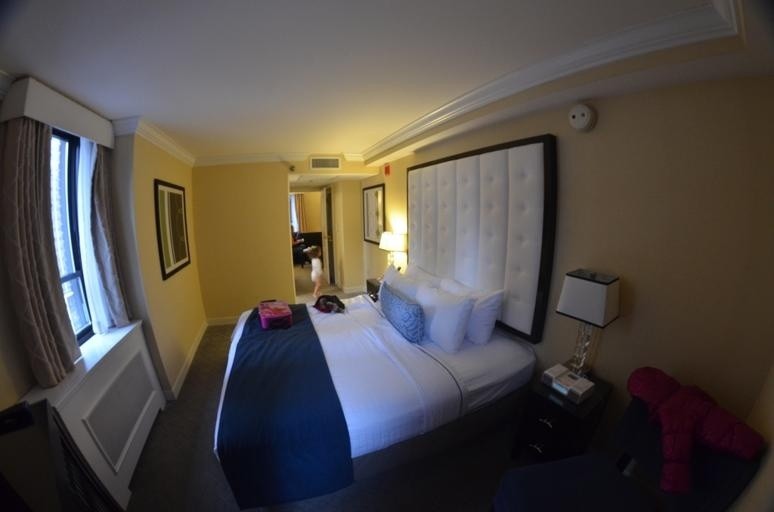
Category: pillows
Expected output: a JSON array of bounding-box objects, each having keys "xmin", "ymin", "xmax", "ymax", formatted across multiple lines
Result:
[
  {"xmin": 438, "ymin": 273, "xmax": 506, "ymax": 349},
  {"xmin": 414, "ymin": 282, "xmax": 478, "ymax": 354},
  {"xmin": 375, "ymin": 278, "xmax": 425, "ymax": 345},
  {"xmin": 403, "ymin": 261, "xmax": 444, "ymax": 288},
  {"xmin": 376, "ymin": 263, "xmax": 423, "ymax": 302}
]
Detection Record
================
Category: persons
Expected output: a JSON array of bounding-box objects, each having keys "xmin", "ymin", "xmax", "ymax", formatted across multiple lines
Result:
[
  {"xmin": 308, "ymin": 246, "xmax": 323, "ymax": 298},
  {"xmin": 291, "ymin": 226, "xmax": 305, "ymax": 245}
]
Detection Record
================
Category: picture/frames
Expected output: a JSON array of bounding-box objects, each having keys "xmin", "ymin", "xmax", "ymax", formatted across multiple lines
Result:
[
  {"xmin": 151, "ymin": 176, "xmax": 191, "ymax": 282},
  {"xmin": 360, "ymin": 183, "xmax": 388, "ymax": 243}
]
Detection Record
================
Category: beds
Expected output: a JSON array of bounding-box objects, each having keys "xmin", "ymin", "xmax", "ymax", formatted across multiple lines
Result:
[{"xmin": 206, "ymin": 290, "xmax": 540, "ymax": 510}]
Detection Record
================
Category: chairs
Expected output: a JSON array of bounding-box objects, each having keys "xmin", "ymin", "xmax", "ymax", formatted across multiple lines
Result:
[{"xmin": 479, "ymin": 391, "xmax": 771, "ymax": 512}]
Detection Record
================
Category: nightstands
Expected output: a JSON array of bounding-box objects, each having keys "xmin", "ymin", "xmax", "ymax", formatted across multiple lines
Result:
[
  {"xmin": 365, "ymin": 276, "xmax": 382, "ymax": 295},
  {"xmin": 513, "ymin": 353, "xmax": 614, "ymax": 461}
]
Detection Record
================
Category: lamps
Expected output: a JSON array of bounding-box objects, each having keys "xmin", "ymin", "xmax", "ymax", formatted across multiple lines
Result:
[
  {"xmin": 377, "ymin": 230, "xmax": 406, "ymax": 265},
  {"xmin": 552, "ymin": 264, "xmax": 623, "ymax": 381}
]
